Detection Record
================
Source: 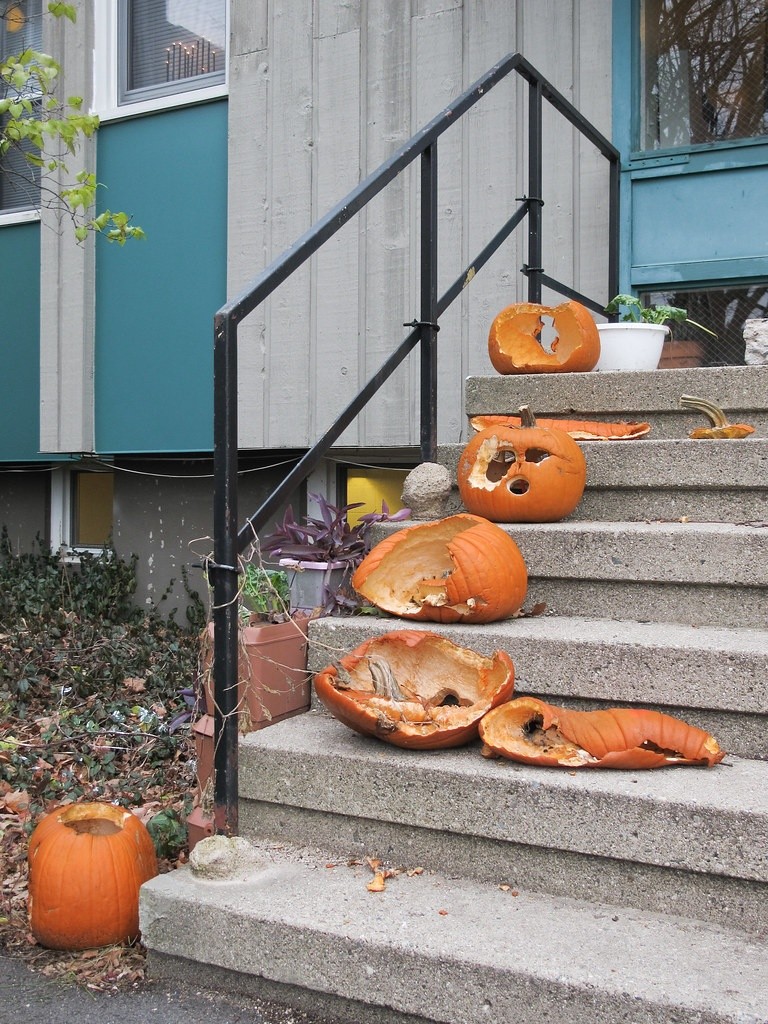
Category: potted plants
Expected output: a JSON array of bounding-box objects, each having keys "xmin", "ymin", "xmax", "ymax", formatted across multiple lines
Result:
[
  {"xmin": 253, "ymin": 489, "xmax": 411, "ymax": 615},
  {"xmin": 659, "ymin": 340, "xmax": 703, "ymax": 368},
  {"xmin": 589, "ymin": 292, "xmax": 688, "ymax": 370}
]
